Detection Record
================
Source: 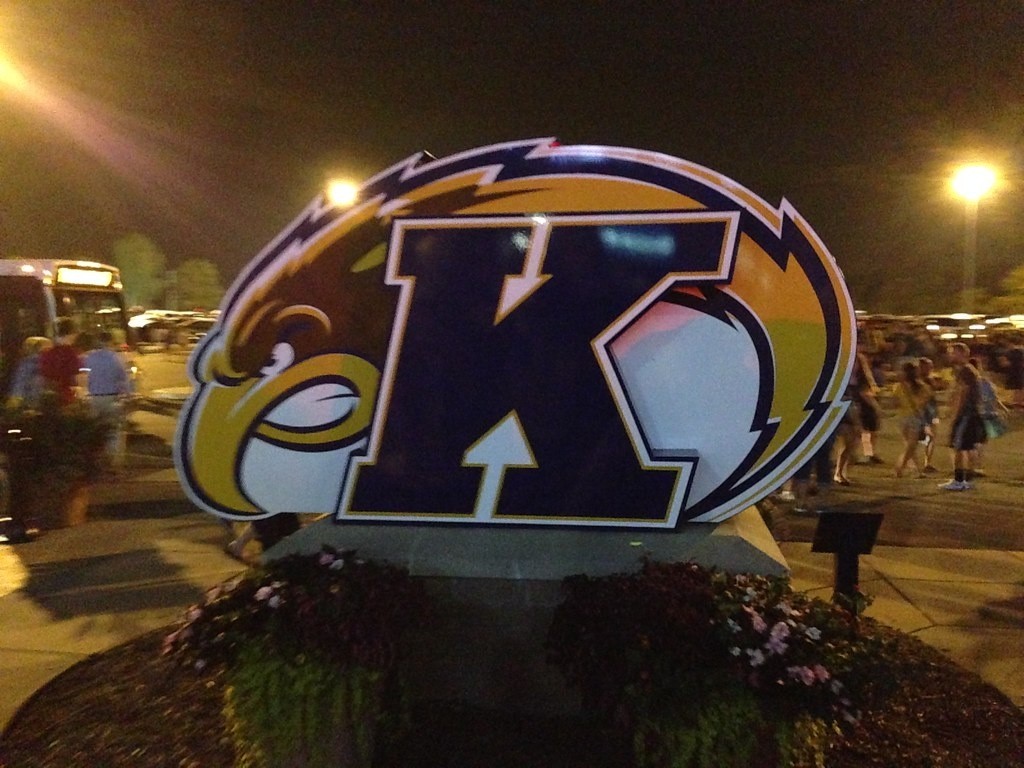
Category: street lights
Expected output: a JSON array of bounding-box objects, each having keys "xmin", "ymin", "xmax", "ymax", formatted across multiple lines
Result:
[{"xmin": 949, "ymin": 160, "xmax": 1000, "ymax": 314}]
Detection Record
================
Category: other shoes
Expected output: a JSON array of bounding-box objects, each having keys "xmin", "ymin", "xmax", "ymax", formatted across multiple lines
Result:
[
  {"xmin": 869, "ymin": 456, "xmax": 883, "ymax": 464},
  {"xmin": 779, "ymin": 489, "xmax": 797, "ymax": 500},
  {"xmin": 795, "ymin": 504, "xmax": 810, "ymax": 512},
  {"xmin": 966, "ymin": 466, "xmax": 986, "ymax": 478},
  {"xmin": 923, "ymin": 464, "xmax": 939, "ymax": 473},
  {"xmin": 849, "ymin": 460, "xmax": 867, "ymax": 466},
  {"xmin": 937, "ymin": 478, "xmax": 971, "ymax": 490},
  {"xmin": 834, "ymin": 474, "xmax": 851, "ymax": 486},
  {"xmin": 816, "ymin": 504, "xmax": 828, "ymax": 513}
]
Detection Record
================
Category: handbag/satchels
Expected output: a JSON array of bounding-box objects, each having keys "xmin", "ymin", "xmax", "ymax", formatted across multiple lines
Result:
[
  {"xmin": 974, "ymin": 415, "xmax": 988, "ymax": 444},
  {"xmin": 918, "ymin": 431, "xmax": 930, "ymax": 447},
  {"xmin": 983, "ymin": 414, "xmax": 1006, "ymax": 439}
]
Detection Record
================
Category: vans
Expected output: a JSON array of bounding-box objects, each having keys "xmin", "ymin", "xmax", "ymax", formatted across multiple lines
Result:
[{"xmin": 0, "ymin": 259, "xmax": 142, "ymax": 399}]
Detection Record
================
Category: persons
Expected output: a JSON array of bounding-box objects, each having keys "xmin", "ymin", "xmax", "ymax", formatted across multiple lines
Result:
[
  {"xmin": 224, "ymin": 513, "xmax": 306, "ymax": 560},
  {"xmin": 773, "ymin": 321, "xmax": 1024, "ymax": 517},
  {"xmin": 11, "ymin": 319, "xmax": 132, "ymax": 523}
]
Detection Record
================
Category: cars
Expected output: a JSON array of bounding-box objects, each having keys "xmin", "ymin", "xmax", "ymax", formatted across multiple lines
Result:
[{"xmin": 125, "ymin": 310, "xmax": 219, "ymax": 344}]
